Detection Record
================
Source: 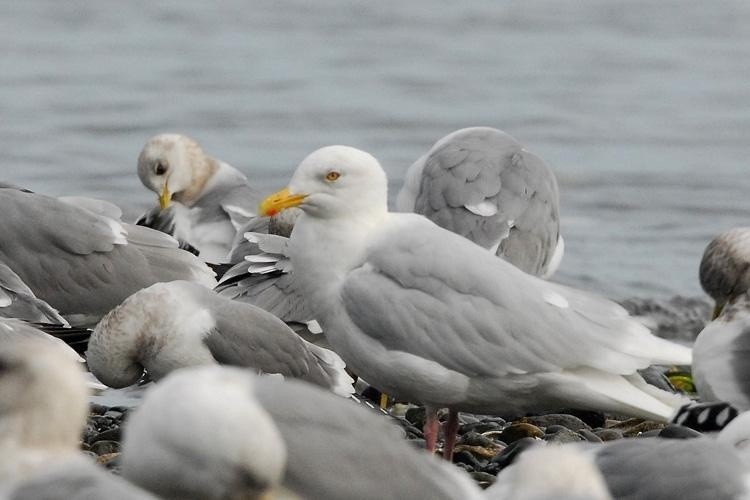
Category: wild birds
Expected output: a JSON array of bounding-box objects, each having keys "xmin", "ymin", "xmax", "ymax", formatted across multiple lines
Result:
[{"xmin": 0, "ymin": 127, "xmax": 750, "ymax": 500}]
